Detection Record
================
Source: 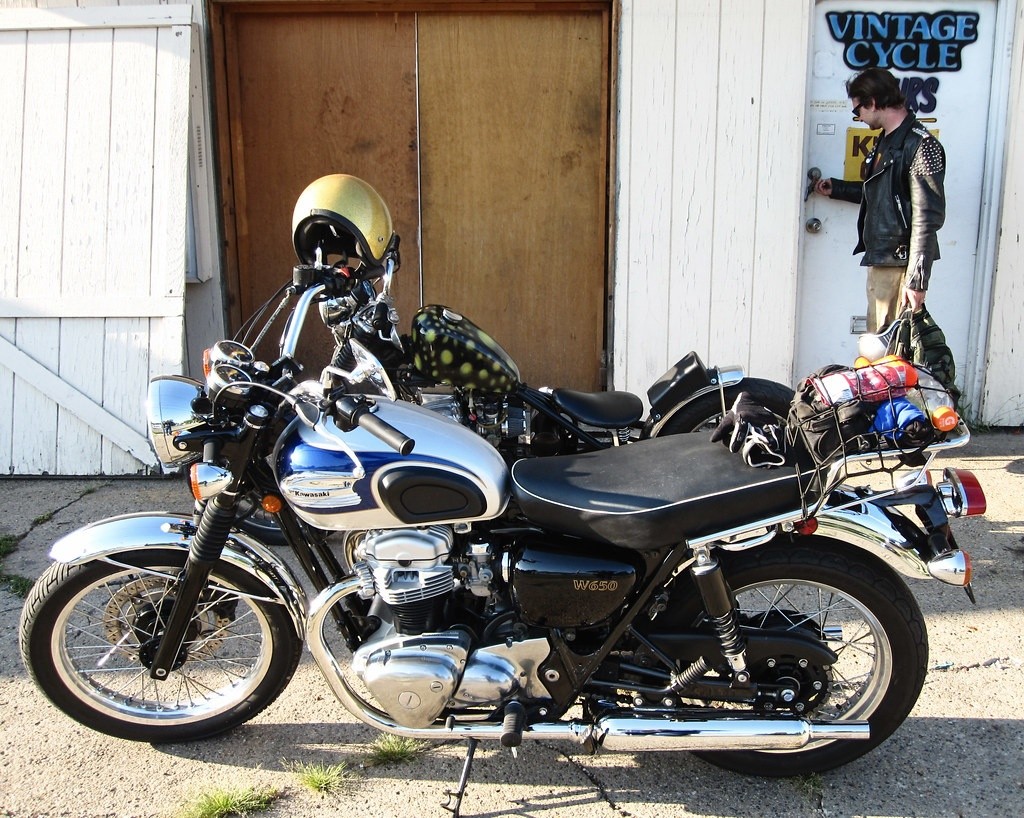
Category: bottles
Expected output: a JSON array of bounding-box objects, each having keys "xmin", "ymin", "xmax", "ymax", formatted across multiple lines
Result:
[{"xmin": 906, "ymin": 362, "xmax": 957, "ymax": 432}]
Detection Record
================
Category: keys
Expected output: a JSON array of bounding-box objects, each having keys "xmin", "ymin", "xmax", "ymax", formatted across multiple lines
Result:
[{"xmin": 805, "ymin": 175, "xmax": 825, "ymax": 201}]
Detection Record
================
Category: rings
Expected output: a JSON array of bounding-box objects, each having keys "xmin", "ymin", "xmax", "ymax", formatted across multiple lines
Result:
[{"xmin": 924, "ymin": 298, "xmax": 925, "ymax": 300}]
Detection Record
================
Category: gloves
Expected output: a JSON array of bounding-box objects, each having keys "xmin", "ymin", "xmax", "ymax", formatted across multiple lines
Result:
[{"xmin": 710, "ymin": 391, "xmax": 788, "ymax": 469}]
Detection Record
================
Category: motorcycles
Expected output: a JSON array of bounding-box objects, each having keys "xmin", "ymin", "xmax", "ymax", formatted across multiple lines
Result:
[{"xmin": 17, "ymin": 232, "xmax": 989, "ymax": 818}]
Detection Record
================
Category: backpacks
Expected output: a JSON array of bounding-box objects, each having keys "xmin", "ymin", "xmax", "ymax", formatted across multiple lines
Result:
[{"xmin": 893, "ymin": 302, "xmax": 961, "ymax": 415}]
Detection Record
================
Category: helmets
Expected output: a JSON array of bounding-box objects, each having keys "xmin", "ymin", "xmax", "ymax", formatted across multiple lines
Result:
[
  {"xmin": 858, "ymin": 318, "xmax": 903, "ymax": 361},
  {"xmin": 292, "ymin": 172, "xmax": 400, "ymax": 273}
]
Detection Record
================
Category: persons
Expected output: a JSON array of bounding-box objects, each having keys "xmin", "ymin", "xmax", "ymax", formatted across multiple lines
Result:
[{"xmin": 814, "ymin": 69, "xmax": 946, "ymax": 333}]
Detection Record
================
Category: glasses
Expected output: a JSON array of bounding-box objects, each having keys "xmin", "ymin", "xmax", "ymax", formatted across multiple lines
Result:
[{"xmin": 851, "ymin": 101, "xmax": 866, "ymax": 117}]
